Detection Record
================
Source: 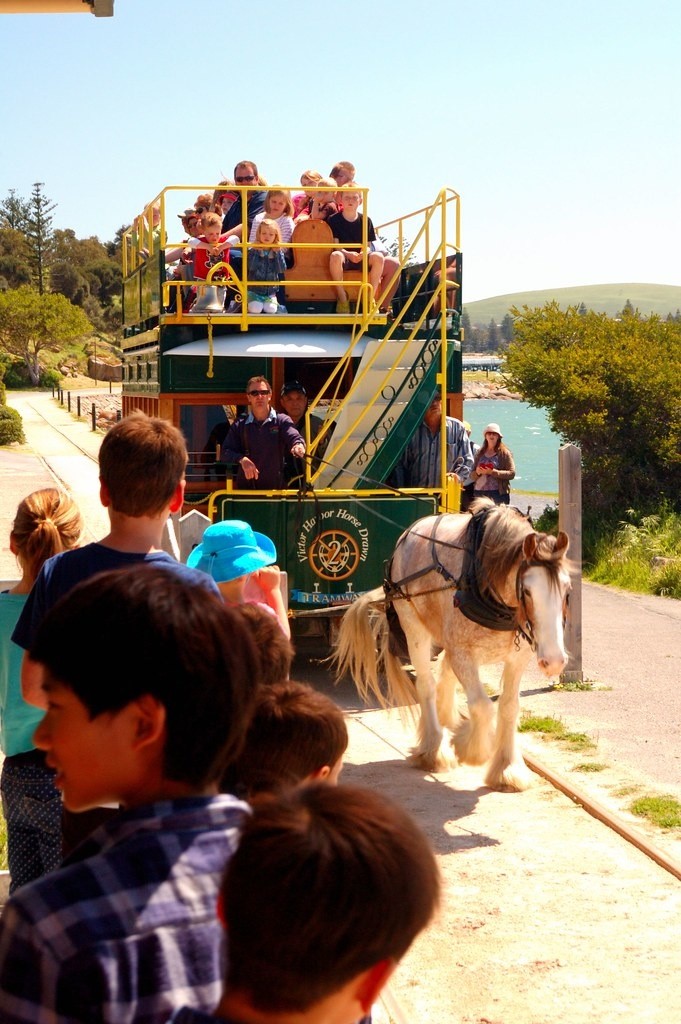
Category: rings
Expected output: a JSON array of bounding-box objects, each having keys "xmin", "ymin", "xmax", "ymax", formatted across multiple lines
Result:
[{"xmin": 254, "ymin": 469, "xmax": 257, "ymax": 473}]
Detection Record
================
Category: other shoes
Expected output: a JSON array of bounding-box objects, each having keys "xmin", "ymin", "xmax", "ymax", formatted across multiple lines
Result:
[{"xmin": 139, "ymin": 247, "xmax": 149, "ymax": 260}]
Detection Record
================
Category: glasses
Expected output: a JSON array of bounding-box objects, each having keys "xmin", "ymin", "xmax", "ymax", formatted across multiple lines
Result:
[
  {"xmin": 195, "ymin": 207, "xmax": 207, "ymax": 214},
  {"xmin": 434, "ymin": 397, "xmax": 441, "ymax": 401},
  {"xmin": 188, "ymin": 222, "xmax": 197, "ymax": 229},
  {"xmin": 237, "ymin": 176, "xmax": 255, "ymax": 182},
  {"xmin": 248, "ymin": 390, "xmax": 270, "ymax": 396}
]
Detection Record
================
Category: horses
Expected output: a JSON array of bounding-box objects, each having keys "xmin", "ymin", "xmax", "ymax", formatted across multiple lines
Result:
[{"xmin": 320, "ymin": 495, "xmax": 572, "ymax": 795}]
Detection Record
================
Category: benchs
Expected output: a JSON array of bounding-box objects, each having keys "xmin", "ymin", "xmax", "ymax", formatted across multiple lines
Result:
[{"xmin": 276, "ymin": 218, "xmax": 382, "ymax": 302}]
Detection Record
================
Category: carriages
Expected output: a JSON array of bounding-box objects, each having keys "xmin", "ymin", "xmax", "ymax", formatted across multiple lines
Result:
[{"xmin": 120, "ymin": 184, "xmax": 574, "ymax": 793}]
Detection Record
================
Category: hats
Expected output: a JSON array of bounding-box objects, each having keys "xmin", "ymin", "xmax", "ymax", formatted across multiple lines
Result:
[
  {"xmin": 484, "ymin": 423, "xmax": 503, "ymax": 437},
  {"xmin": 186, "ymin": 518, "xmax": 277, "ymax": 584},
  {"xmin": 281, "ymin": 380, "xmax": 307, "ymax": 397},
  {"xmin": 463, "ymin": 421, "xmax": 471, "ymax": 433},
  {"xmin": 176, "ymin": 208, "xmax": 195, "ymax": 218},
  {"xmin": 216, "ymin": 191, "xmax": 238, "ymax": 207}
]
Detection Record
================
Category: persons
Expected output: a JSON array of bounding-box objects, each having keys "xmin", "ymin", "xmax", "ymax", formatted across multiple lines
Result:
[
  {"xmin": 459, "ymin": 420, "xmax": 482, "ymax": 514},
  {"xmin": 389, "ymin": 392, "xmax": 474, "ymax": 505},
  {"xmin": 131, "ymin": 161, "xmax": 402, "ymax": 315},
  {"xmin": 187, "ymin": 521, "xmax": 291, "ymax": 641},
  {"xmin": 279, "ymin": 381, "xmax": 333, "ymax": 489},
  {"xmin": 11, "ymin": 413, "xmax": 225, "ymax": 711},
  {"xmin": 222, "ymin": 376, "xmax": 306, "ymax": 489},
  {"xmin": 228, "ymin": 602, "xmax": 292, "ymax": 685},
  {"xmin": 173, "ymin": 785, "xmax": 439, "ymax": 1024},
  {"xmin": 224, "ymin": 682, "xmax": 347, "ymax": 801},
  {"xmin": 0, "ymin": 568, "xmax": 259, "ymax": 1024},
  {"xmin": 0, "ymin": 488, "xmax": 85, "ymax": 898},
  {"xmin": 470, "ymin": 423, "xmax": 515, "ymax": 508}
]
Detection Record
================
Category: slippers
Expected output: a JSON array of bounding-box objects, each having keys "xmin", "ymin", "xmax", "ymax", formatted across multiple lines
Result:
[
  {"xmin": 371, "ymin": 298, "xmax": 379, "ymax": 313},
  {"xmin": 336, "ymin": 290, "xmax": 350, "ymax": 314}
]
